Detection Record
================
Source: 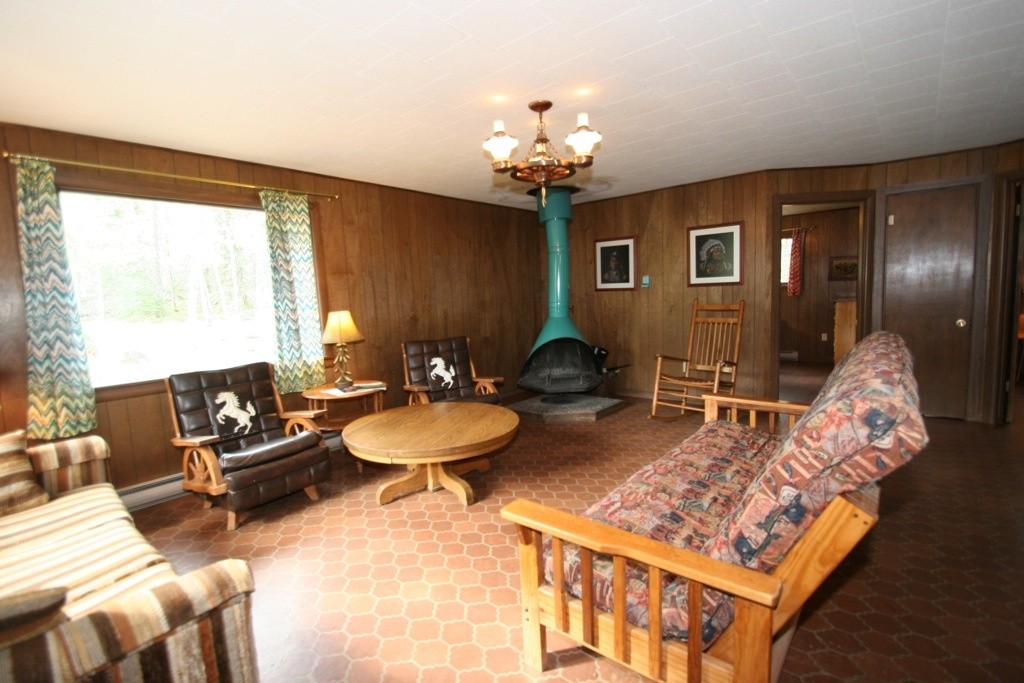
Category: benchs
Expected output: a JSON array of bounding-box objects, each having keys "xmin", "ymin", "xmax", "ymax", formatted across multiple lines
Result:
[{"xmin": 500, "ymin": 330, "xmax": 931, "ymax": 683}]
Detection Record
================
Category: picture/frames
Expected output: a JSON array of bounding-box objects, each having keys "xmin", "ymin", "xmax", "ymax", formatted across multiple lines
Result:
[
  {"xmin": 593, "ymin": 235, "xmax": 637, "ymax": 292},
  {"xmin": 685, "ymin": 220, "xmax": 744, "ymax": 288}
]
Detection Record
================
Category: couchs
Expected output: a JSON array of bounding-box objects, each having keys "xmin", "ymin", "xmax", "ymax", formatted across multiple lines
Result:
[
  {"xmin": 165, "ymin": 361, "xmax": 332, "ymax": 531},
  {"xmin": 402, "ymin": 335, "xmax": 505, "ymax": 408},
  {"xmin": 0, "ymin": 436, "xmax": 261, "ymax": 683}
]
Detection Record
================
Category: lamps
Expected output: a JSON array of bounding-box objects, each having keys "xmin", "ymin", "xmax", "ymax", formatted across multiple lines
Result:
[
  {"xmin": 320, "ymin": 311, "xmax": 364, "ymax": 389},
  {"xmin": 481, "ymin": 101, "xmax": 603, "ymax": 208}
]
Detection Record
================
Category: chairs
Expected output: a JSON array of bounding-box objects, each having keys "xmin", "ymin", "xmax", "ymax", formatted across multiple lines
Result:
[{"xmin": 651, "ymin": 299, "xmax": 744, "ymax": 422}]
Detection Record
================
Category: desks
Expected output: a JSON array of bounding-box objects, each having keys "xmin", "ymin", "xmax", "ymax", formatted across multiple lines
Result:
[
  {"xmin": 305, "ymin": 381, "xmax": 388, "ymax": 473},
  {"xmin": 342, "ymin": 401, "xmax": 519, "ymax": 505}
]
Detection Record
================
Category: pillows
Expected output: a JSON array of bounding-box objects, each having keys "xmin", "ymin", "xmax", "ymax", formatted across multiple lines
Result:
[
  {"xmin": 0, "ymin": 429, "xmax": 49, "ymax": 514},
  {"xmin": 0, "ymin": 586, "xmax": 71, "ymax": 649}
]
